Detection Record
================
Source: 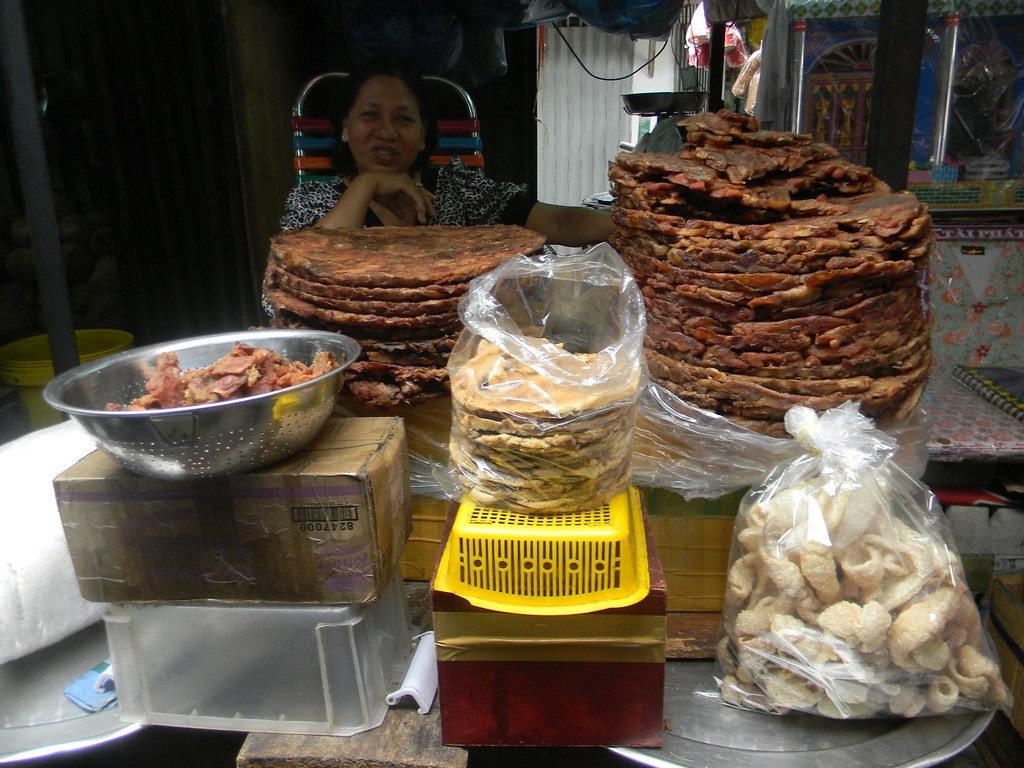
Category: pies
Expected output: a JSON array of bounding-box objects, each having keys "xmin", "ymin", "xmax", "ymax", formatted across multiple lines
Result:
[{"xmin": 447, "ymin": 335, "xmax": 641, "ymax": 517}]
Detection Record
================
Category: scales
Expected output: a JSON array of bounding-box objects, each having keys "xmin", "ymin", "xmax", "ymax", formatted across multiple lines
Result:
[{"xmin": 580, "ymin": 91, "xmax": 707, "ymax": 211}]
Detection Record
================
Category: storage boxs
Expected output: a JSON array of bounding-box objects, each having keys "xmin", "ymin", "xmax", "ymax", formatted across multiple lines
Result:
[{"xmin": 55, "ymin": 416, "xmax": 668, "ymax": 752}]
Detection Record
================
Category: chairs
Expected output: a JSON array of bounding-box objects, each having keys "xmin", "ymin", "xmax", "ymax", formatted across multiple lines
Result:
[{"xmin": 289, "ymin": 73, "xmax": 485, "ymax": 189}]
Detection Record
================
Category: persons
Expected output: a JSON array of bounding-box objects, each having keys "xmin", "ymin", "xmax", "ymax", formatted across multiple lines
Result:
[{"xmin": 259, "ymin": 55, "xmax": 613, "ymax": 319}]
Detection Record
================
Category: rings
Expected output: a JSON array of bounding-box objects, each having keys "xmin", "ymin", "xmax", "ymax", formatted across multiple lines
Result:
[{"xmin": 413, "ymin": 177, "xmax": 423, "ymax": 191}]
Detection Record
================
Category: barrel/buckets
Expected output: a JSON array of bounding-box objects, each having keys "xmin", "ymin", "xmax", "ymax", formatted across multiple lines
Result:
[{"xmin": 1, "ymin": 329, "xmax": 136, "ymax": 431}]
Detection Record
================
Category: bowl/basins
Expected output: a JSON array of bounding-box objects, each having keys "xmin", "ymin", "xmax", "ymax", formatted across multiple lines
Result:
[{"xmin": 43, "ymin": 329, "xmax": 361, "ymax": 480}]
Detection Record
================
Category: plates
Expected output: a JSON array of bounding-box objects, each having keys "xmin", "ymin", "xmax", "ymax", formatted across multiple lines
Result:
[{"xmin": 618, "ymin": 91, "xmax": 708, "ymax": 113}]
